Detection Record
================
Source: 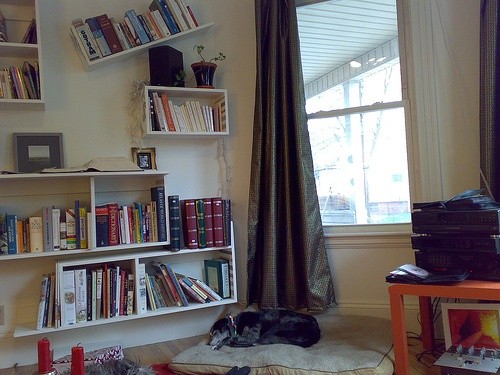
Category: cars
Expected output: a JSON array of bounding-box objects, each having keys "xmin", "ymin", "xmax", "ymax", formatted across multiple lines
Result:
[{"xmin": 316, "ymin": 160, "xmax": 413, "ymax": 225}]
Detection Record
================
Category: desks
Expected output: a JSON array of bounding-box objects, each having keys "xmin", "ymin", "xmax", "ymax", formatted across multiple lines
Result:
[{"xmin": 389, "ymin": 280, "xmax": 500, "ymax": 375}]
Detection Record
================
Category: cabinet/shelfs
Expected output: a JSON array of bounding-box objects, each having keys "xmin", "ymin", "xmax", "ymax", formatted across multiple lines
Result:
[
  {"xmin": 0, "ymin": 0, "xmax": 45, "ymax": 103},
  {"xmin": 0, "ymin": 172, "xmax": 238, "ymax": 338},
  {"xmin": 140, "ymin": 86, "xmax": 229, "ymax": 138}
]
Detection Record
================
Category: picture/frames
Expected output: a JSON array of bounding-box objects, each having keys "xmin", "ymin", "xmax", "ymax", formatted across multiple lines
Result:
[
  {"xmin": 131, "ymin": 147, "xmax": 157, "ymax": 170},
  {"xmin": 13, "ymin": 133, "xmax": 64, "ymax": 173},
  {"xmin": 441, "ymin": 303, "xmax": 500, "ymax": 351}
]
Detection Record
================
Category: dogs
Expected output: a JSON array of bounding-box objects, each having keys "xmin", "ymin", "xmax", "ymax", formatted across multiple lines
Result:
[{"xmin": 206, "ymin": 308, "xmax": 322, "ymax": 351}]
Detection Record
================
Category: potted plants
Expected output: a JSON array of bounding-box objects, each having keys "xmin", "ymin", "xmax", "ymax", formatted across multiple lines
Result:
[{"xmin": 191, "ymin": 44, "xmax": 226, "ymax": 86}]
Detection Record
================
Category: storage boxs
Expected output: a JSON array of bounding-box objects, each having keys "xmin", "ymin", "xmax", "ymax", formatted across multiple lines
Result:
[{"xmin": 53, "ymin": 345, "xmax": 124, "ymax": 375}]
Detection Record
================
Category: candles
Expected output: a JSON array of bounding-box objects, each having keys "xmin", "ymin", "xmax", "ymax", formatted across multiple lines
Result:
[
  {"xmin": 71, "ymin": 342, "xmax": 85, "ymax": 375},
  {"xmin": 38, "ymin": 337, "xmax": 51, "ymax": 373}
]
